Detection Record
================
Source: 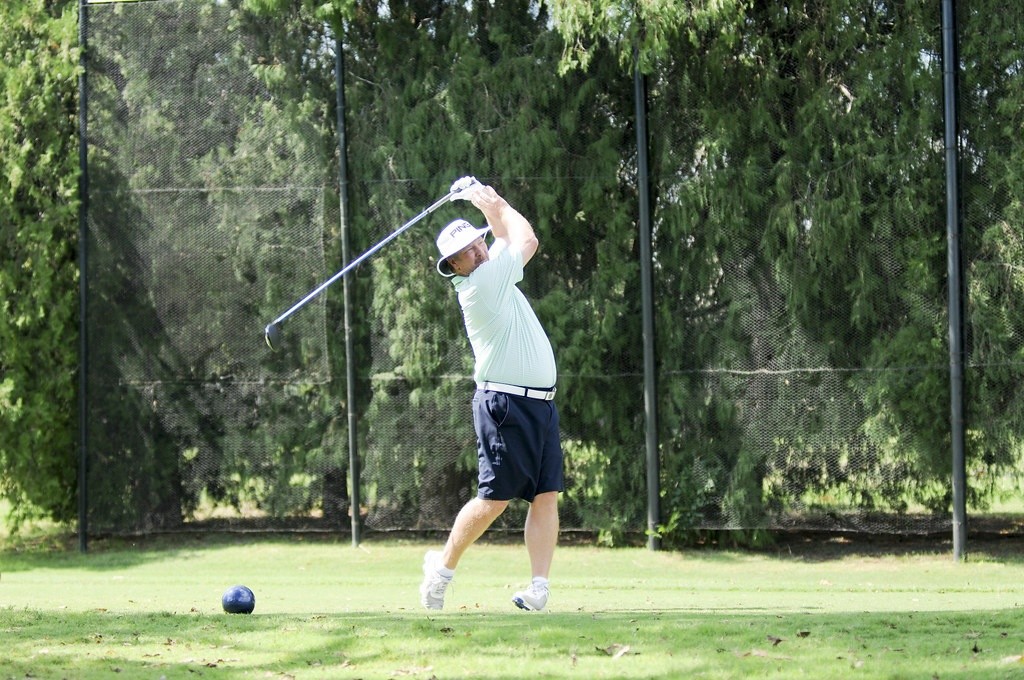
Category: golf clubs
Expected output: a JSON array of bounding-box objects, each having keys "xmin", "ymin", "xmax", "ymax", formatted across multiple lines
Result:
[{"xmin": 264, "ymin": 176, "xmax": 476, "ymax": 354}]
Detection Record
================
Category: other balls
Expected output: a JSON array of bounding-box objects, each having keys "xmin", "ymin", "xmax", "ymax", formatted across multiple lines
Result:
[{"xmin": 222, "ymin": 584, "xmax": 255, "ymax": 615}]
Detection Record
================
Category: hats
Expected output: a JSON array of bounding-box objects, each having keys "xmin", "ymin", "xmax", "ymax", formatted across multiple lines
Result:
[{"xmin": 433, "ymin": 218, "xmax": 491, "ymax": 278}]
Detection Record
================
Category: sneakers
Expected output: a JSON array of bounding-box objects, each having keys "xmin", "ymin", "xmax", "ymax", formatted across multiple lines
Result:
[
  {"xmin": 511, "ymin": 577, "xmax": 549, "ymax": 612},
  {"xmin": 422, "ymin": 548, "xmax": 454, "ymax": 611}
]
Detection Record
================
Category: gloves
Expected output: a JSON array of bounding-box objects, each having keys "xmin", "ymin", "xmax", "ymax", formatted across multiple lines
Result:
[{"xmin": 448, "ymin": 175, "xmax": 486, "ymax": 203}]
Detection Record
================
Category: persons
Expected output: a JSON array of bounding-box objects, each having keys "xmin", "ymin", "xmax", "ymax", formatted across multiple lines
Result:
[{"xmin": 424, "ymin": 173, "xmax": 572, "ymax": 616}]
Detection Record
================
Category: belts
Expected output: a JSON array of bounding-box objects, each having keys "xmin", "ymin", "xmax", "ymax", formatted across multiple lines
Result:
[{"xmin": 474, "ymin": 380, "xmax": 557, "ymax": 402}]
